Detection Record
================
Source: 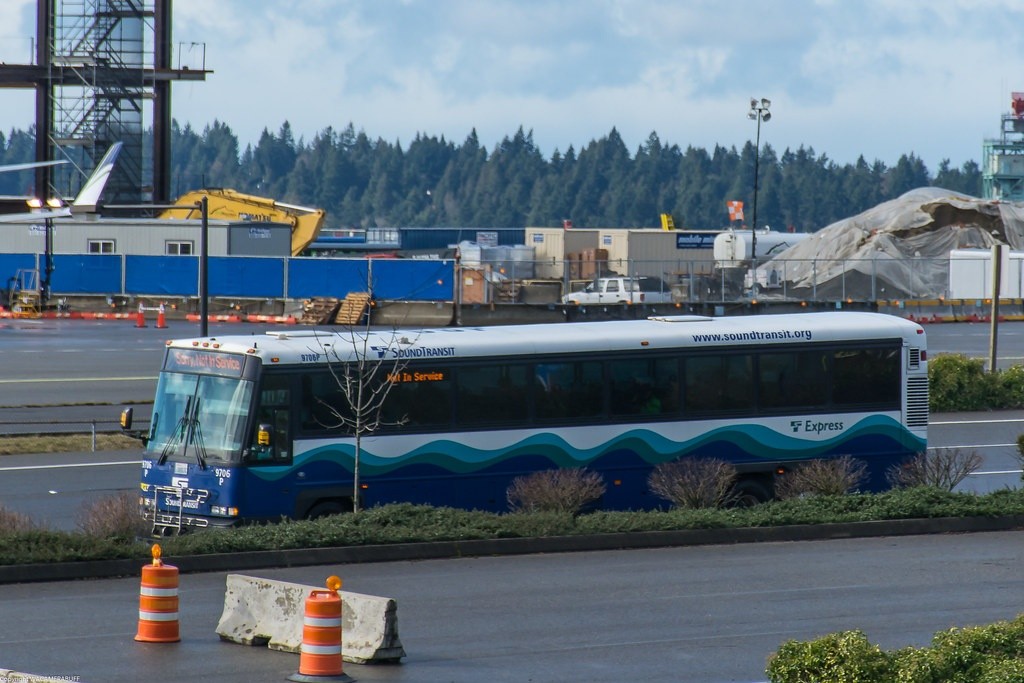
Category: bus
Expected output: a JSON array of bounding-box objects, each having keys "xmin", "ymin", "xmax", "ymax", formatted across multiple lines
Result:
[{"xmin": 120, "ymin": 311, "xmax": 930, "ymax": 538}]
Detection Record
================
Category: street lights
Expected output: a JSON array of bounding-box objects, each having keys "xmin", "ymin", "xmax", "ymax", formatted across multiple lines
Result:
[
  {"xmin": 746, "ymin": 96, "xmax": 771, "ymax": 268},
  {"xmin": 26, "ymin": 197, "xmax": 63, "ymax": 299}
]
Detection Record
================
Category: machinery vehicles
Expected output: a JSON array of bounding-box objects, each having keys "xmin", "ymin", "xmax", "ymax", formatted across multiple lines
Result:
[{"xmin": 714, "ymin": 227, "xmax": 811, "ymax": 276}]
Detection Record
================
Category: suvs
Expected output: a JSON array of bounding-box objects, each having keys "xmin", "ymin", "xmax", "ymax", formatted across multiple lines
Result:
[{"xmin": 559, "ymin": 275, "xmax": 674, "ymax": 317}]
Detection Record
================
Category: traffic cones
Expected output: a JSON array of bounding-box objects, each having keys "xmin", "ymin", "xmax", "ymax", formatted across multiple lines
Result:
[
  {"xmin": 154, "ymin": 302, "xmax": 169, "ymax": 327},
  {"xmin": 133, "ymin": 300, "xmax": 149, "ymax": 328}
]
